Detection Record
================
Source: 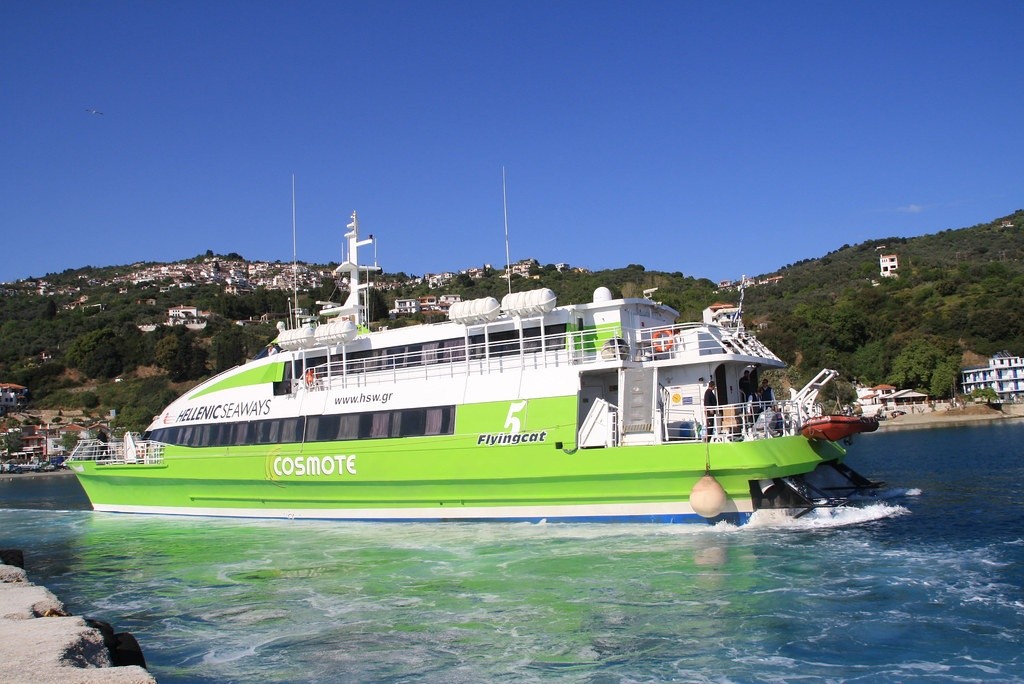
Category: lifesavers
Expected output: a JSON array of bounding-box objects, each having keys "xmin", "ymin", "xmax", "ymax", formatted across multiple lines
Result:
[
  {"xmin": 653, "ymin": 328, "xmax": 673, "ymax": 352},
  {"xmin": 306, "ymin": 370, "xmax": 318, "ymax": 386}
]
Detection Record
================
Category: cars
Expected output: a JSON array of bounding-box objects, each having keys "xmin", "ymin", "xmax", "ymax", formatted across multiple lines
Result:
[{"xmin": 0, "ymin": 459, "xmax": 56, "ymax": 473}]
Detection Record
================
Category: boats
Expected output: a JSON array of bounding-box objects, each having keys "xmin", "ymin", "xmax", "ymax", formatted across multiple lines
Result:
[
  {"xmin": 59, "ymin": 171, "xmax": 927, "ymax": 536},
  {"xmin": 801, "ymin": 414, "xmax": 878, "ymax": 442}
]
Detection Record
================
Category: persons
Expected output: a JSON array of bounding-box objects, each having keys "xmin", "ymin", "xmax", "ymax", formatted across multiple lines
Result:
[
  {"xmin": 265, "ymin": 343, "xmax": 278, "ymax": 356},
  {"xmin": 703, "ymin": 381, "xmax": 717, "ymax": 443},
  {"xmin": 96, "ymin": 428, "xmax": 108, "ymax": 460},
  {"xmin": 739, "ymin": 370, "xmax": 776, "ymax": 428}
]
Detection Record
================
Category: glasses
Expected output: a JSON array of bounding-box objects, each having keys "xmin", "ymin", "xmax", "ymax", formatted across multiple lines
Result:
[{"xmin": 762, "ymin": 382, "xmax": 767, "ymax": 384}]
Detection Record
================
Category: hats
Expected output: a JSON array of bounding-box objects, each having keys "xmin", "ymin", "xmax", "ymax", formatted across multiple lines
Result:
[{"xmin": 267, "ymin": 343, "xmax": 272, "ymax": 346}]
